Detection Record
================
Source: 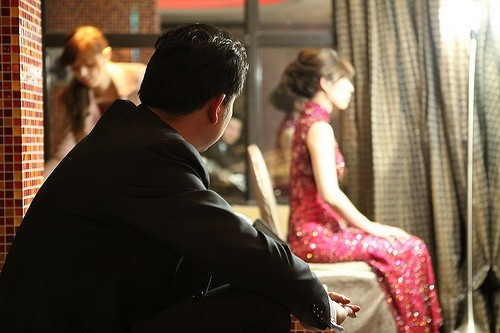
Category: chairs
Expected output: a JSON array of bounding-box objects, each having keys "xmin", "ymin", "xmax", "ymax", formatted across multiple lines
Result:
[{"xmin": 247, "ymin": 144, "xmax": 397, "ymax": 333}]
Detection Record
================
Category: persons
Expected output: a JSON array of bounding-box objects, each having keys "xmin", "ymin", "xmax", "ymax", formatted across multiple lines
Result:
[
  {"xmin": 268, "ymin": 46, "xmax": 445, "ymax": 333},
  {"xmin": 0, "ymin": 21, "xmax": 361, "ymax": 333},
  {"xmin": 269, "ymin": 82, "xmax": 307, "ymax": 199},
  {"xmin": 43, "ymin": 26, "xmax": 148, "ymax": 183},
  {"xmin": 201, "ymin": 115, "xmax": 249, "ymax": 198}
]
